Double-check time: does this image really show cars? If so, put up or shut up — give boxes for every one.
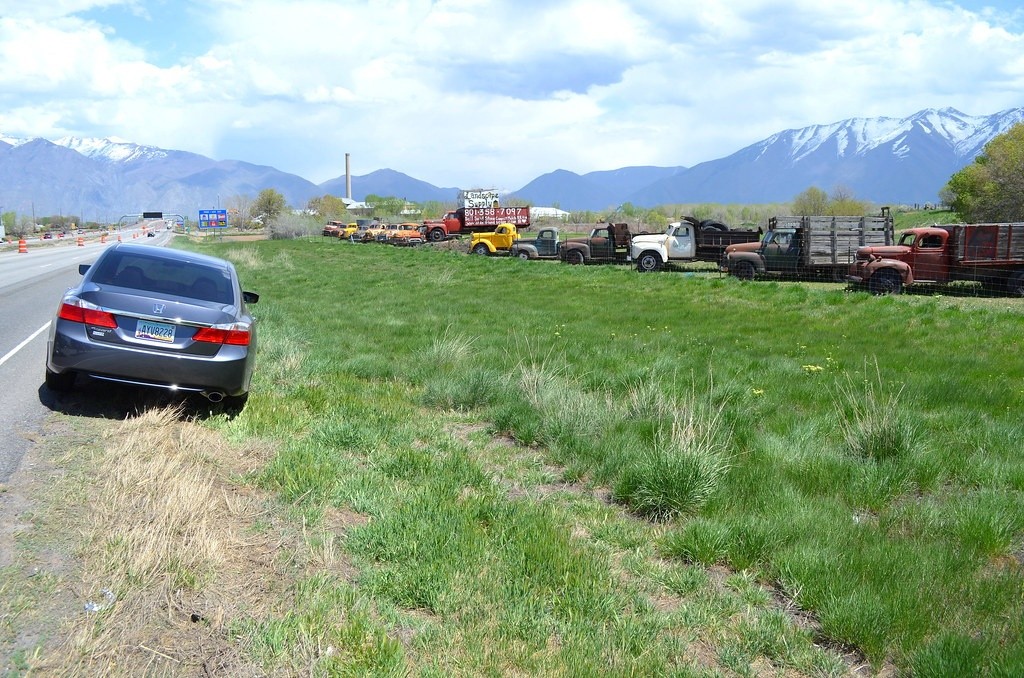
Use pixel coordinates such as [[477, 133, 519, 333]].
[[77, 230, 83, 234], [135, 221, 140, 224], [99, 226, 106, 231], [155, 229, 160, 232], [45, 241, 259, 416], [59, 232, 64, 237], [44, 233, 51, 239], [147, 232, 155, 237], [113, 226, 119, 230], [165, 219, 178, 229]]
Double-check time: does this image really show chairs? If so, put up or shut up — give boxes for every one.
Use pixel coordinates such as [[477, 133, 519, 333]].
[[114, 265, 146, 288], [187, 276, 218, 302]]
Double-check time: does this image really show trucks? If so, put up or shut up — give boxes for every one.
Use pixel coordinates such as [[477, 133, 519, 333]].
[[465, 223, 522, 257], [718, 207, 895, 283], [322, 221, 428, 246], [846, 223, 1024, 298], [508, 227, 563, 260], [626, 216, 764, 272], [423, 189, 531, 242], [558, 223, 666, 265]]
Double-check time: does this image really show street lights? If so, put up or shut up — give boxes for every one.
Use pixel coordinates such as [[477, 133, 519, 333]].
[[217, 192, 224, 242]]
